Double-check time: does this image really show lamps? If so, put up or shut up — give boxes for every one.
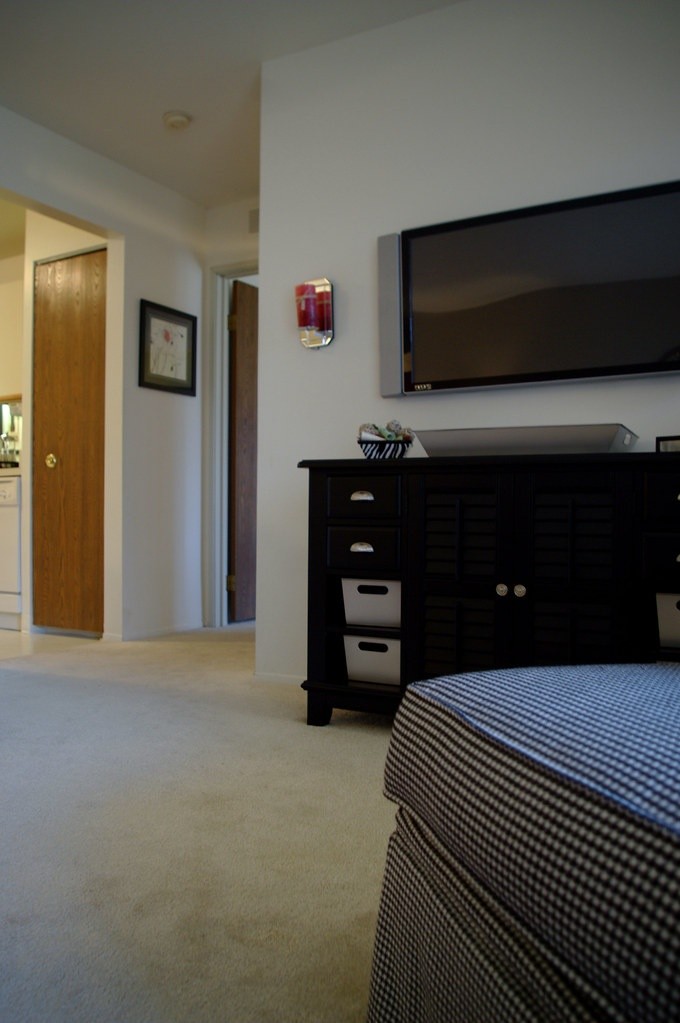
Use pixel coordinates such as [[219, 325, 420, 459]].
[[296, 277, 336, 350]]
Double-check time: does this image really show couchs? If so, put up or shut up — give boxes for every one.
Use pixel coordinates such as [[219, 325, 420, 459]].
[[362, 661, 680, 1023]]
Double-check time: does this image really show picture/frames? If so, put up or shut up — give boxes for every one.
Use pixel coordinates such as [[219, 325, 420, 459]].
[[138, 298, 198, 397]]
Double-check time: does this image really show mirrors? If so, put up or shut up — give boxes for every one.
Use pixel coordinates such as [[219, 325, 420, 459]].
[[0, 394, 22, 455]]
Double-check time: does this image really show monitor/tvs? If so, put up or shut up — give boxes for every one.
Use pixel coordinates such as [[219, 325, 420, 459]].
[[399, 179, 680, 396]]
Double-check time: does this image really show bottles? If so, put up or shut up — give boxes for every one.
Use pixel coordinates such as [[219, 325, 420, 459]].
[[0, 432, 19, 467]]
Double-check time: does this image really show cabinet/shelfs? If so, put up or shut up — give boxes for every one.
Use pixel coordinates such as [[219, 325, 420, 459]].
[[298, 453, 680, 727], [0, 476, 20, 631]]
